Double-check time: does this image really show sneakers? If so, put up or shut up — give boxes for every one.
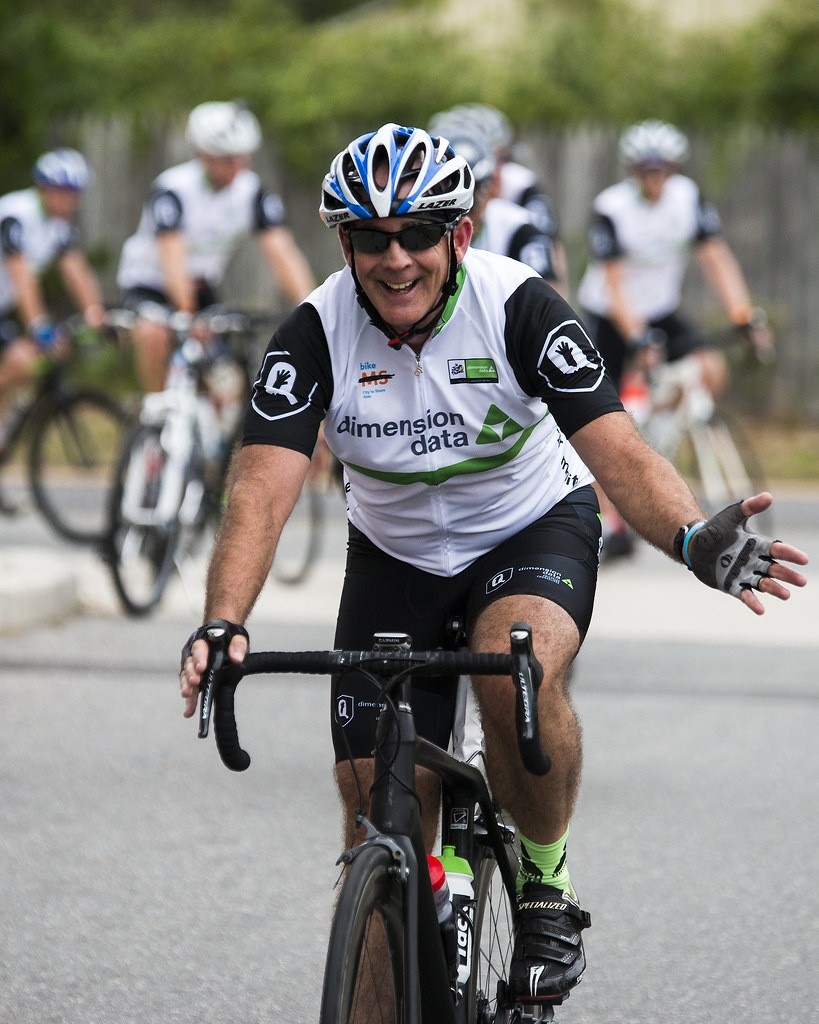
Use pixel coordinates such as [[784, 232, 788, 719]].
[[509, 880, 593, 1004]]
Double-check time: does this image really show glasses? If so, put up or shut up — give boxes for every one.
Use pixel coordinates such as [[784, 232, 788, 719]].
[[341, 213, 466, 256]]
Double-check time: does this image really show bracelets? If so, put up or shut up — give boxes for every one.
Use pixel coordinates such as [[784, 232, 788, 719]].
[[674, 518, 705, 567]]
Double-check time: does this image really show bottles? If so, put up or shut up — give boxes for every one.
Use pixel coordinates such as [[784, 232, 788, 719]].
[[422, 854, 462, 981], [432, 844, 474, 984]]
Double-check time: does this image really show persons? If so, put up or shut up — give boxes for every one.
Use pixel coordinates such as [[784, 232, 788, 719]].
[[0, 148, 103, 394], [578, 121, 767, 561], [115, 101, 318, 472], [429, 103, 570, 300], [178, 122, 808, 1024]]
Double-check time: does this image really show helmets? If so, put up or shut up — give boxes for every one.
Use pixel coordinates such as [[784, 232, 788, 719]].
[[318, 124, 474, 226], [187, 99, 262, 159], [424, 134, 498, 194], [31, 149, 91, 191], [617, 120, 691, 171], [453, 102, 517, 163]]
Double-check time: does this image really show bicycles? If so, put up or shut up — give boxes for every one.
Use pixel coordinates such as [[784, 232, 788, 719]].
[[104, 299, 284, 617], [196, 618, 569, 1023], [1, 333, 133, 546], [640, 320, 774, 542], [229, 314, 326, 585]]
